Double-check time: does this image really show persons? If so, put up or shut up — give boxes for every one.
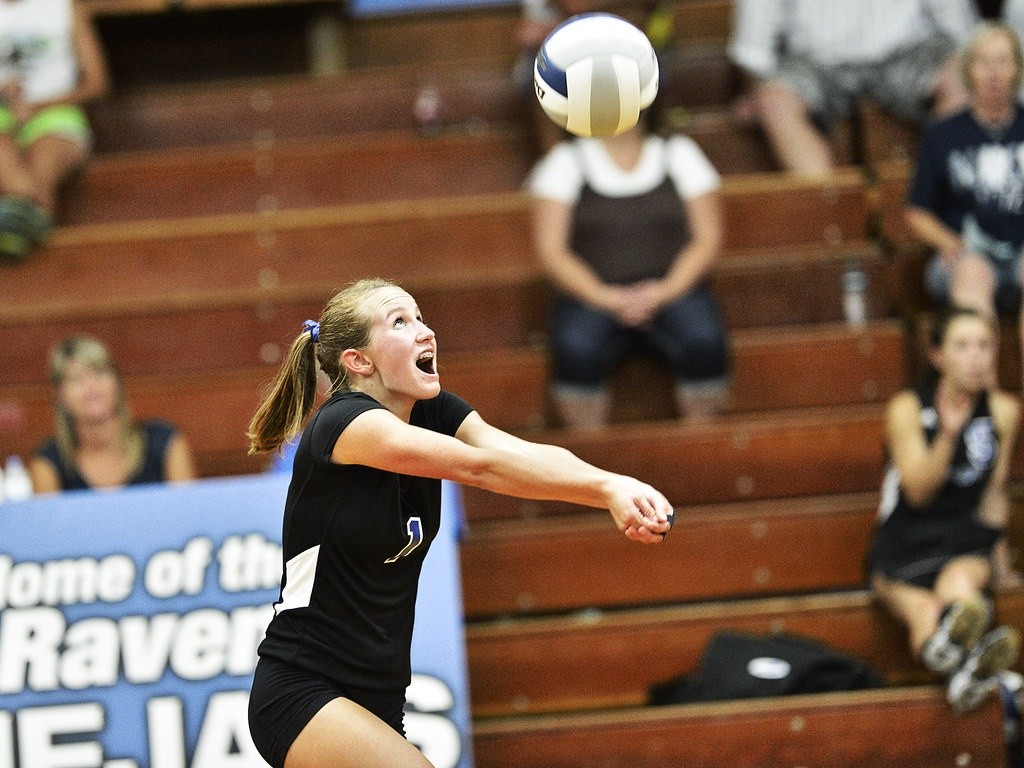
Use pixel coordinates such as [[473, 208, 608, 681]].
[[0, 0, 112, 264], [242, 272, 676, 768], [898, 22, 1024, 351], [24, 331, 200, 495], [861, 306, 1024, 715], [501, 0, 731, 432], [719, 0, 984, 173]]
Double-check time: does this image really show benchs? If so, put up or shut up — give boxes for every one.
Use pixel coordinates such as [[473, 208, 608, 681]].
[[0, 34, 1024, 768]]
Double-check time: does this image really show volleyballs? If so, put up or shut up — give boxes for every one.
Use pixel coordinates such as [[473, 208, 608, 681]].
[[531, 10, 660, 139]]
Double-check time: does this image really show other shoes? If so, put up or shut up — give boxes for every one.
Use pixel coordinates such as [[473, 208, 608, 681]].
[[947, 628, 1019, 713], [0, 196, 51, 258], [914, 596, 991, 675]]
